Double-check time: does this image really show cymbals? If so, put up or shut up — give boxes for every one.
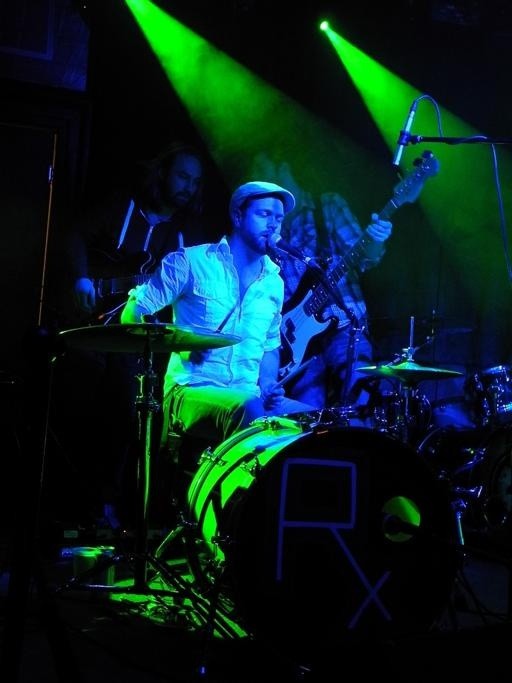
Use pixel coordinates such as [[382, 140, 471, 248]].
[[58, 323, 234, 353], [356, 362, 464, 381]]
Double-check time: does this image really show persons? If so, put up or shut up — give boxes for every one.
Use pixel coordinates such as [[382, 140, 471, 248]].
[[75, 148, 203, 312], [251, 147, 392, 427], [119, 179, 319, 444]]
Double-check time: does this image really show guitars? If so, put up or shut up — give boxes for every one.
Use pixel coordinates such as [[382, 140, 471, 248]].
[[29, 252, 152, 320], [280, 151, 440, 379]]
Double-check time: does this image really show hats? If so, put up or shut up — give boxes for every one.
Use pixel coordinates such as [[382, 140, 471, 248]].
[[229, 181, 296, 213]]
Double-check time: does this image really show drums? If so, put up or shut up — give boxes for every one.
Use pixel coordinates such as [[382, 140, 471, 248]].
[[375, 392, 479, 478], [187, 416, 419, 591], [464, 366, 511, 425]]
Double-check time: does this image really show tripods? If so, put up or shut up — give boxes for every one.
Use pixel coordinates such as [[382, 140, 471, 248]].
[[54, 348, 245, 638]]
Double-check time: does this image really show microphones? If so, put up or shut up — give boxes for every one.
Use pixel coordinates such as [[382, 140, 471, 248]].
[[393, 99, 419, 167], [382, 515, 432, 545], [267, 233, 321, 271]]
